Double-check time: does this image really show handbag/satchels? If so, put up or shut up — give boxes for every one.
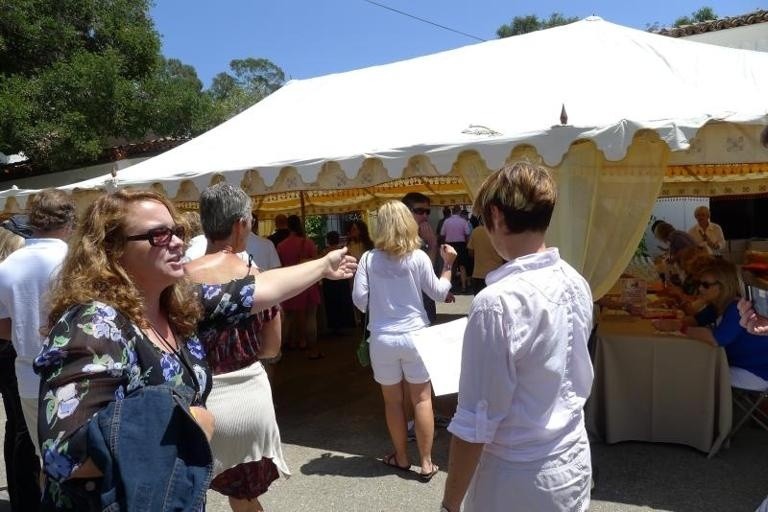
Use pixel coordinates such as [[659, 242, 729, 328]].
[[355, 338, 370, 366]]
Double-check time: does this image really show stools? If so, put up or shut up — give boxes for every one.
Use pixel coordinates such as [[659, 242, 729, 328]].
[[722, 365, 767, 445]]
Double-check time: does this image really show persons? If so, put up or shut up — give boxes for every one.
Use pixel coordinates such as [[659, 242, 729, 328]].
[[597, 205, 766, 402], [352, 194, 504, 480], [438, 159, 595, 512], [0, 182, 358, 511]]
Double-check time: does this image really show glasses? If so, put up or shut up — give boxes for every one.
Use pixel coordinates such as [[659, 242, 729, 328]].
[[696, 279, 717, 289], [117, 226, 186, 247], [412, 207, 431, 215]]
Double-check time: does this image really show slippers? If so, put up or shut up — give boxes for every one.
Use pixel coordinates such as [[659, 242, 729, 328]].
[[419, 465, 438, 480], [382, 455, 411, 469]]
